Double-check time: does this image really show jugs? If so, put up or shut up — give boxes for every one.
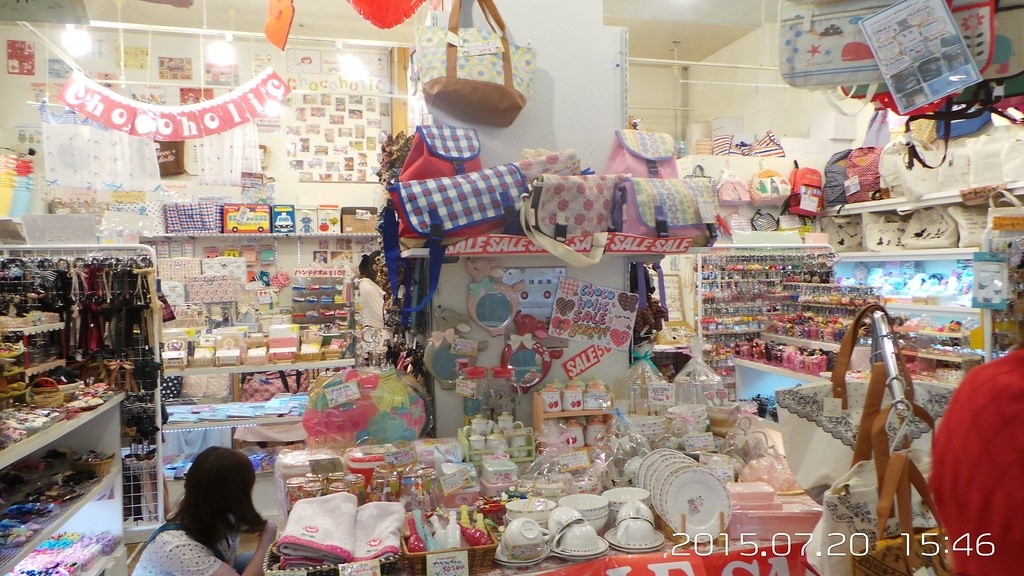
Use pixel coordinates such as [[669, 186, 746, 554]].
[[489, 366, 522, 414], [462, 366, 488, 415]]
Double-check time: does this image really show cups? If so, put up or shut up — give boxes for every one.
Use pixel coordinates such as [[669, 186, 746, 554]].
[[285, 465, 441, 511], [509, 435, 528, 458], [541, 375, 606, 411], [500, 500, 655, 559], [469, 435, 485, 462], [561, 417, 606, 447]]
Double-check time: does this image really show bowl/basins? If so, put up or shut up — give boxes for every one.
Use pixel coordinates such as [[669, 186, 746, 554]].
[[631, 403, 709, 446], [504, 487, 650, 530]]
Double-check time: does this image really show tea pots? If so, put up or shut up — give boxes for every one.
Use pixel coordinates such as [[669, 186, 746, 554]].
[[464, 414, 494, 439], [486, 429, 508, 457], [489, 411, 523, 434]]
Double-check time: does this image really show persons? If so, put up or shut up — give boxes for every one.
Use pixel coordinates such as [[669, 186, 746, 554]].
[[359, 251, 386, 326], [132, 447, 276, 576]]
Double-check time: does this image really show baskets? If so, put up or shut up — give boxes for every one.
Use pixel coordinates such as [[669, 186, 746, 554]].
[[29, 378, 63, 408], [400, 520, 498, 576], [70, 458, 112, 477], [262, 540, 402, 576]]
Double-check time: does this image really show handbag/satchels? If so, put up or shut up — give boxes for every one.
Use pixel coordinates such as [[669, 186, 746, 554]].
[[803, 362, 943, 576], [775, 303, 960, 506], [381, 0, 1024, 314], [239, 370, 308, 402], [850, 452, 954, 576]]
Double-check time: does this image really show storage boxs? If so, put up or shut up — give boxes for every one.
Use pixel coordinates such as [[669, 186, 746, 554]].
[[185, 275, 237, 304], [222, 203, 377, 232]]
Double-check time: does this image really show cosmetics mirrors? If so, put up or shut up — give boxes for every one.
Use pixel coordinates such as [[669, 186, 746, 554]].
[[423, 305, 488, 390], [501, 311, 562, 394], [465, 258, 525, 336]]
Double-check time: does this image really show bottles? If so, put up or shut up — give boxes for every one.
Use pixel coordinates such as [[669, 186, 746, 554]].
[[407, 505, 493, 552]]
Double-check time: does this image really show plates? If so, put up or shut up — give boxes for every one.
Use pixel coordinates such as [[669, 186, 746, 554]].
[[637, 449, 732, 542], [493, 526, 666, 567]]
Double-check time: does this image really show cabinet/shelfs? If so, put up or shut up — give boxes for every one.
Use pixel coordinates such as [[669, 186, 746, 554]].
[[733, 181, 1023, 419], [0, 388, 128, 576]]
